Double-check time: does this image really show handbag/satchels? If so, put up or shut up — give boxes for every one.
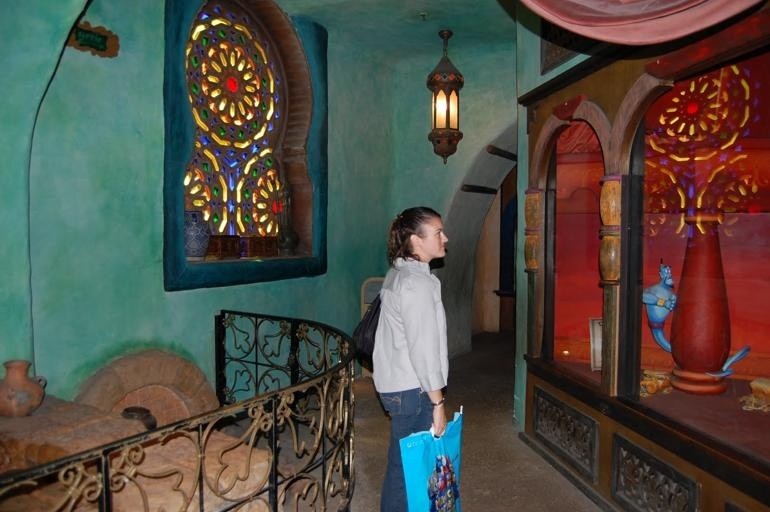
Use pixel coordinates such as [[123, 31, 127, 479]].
[[352, 292, 382, 373]]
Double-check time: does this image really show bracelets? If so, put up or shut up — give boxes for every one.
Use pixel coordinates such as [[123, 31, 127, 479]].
[[431, 397, 445, 406]]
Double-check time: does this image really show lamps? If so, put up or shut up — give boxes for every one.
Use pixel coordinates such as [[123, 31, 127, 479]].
[[427, 27, 464, 168]]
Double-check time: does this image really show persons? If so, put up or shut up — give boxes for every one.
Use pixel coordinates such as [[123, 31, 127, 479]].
[[373, 206, 449, 512]]
